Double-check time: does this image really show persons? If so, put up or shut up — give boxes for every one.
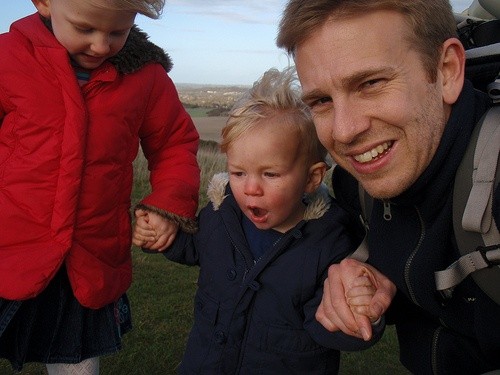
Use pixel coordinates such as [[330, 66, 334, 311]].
[[274, 0, 500, 375], [0, 0, 204, 375], [129, 62, 390, 374]]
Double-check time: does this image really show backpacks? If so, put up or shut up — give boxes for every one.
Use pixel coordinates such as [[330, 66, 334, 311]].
[[357, 2, 500, 309]]
